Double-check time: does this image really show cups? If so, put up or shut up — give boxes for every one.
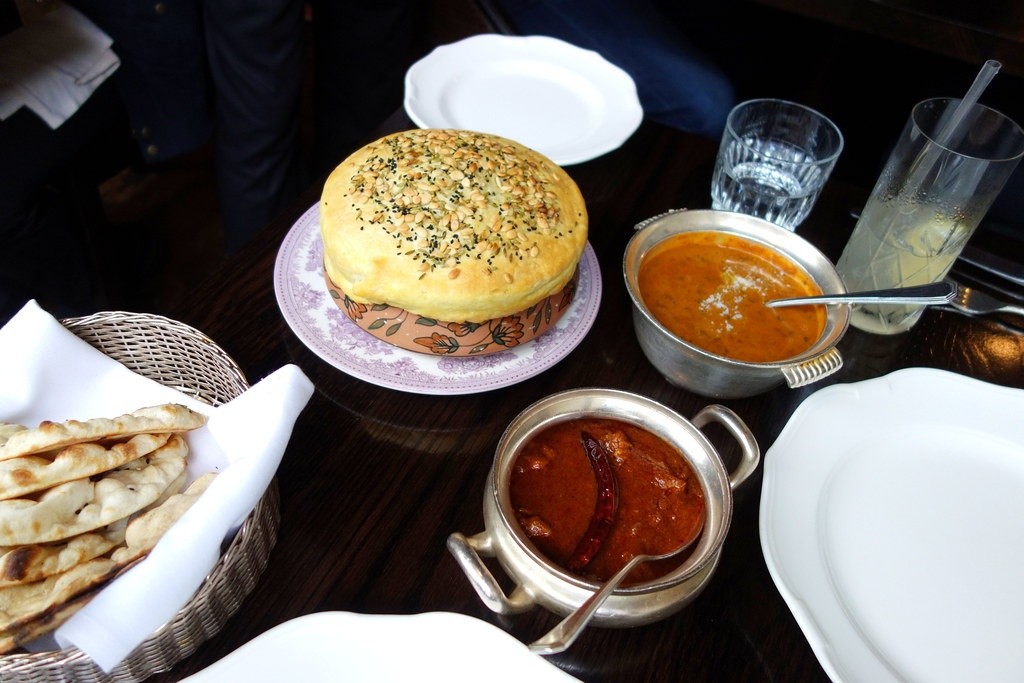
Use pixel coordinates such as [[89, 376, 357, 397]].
[[835, 96, 1024, 336], [709, 97, 845, 232]]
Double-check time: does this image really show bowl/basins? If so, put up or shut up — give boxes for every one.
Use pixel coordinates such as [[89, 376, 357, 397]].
[[621, 205, 853, 397], [445, 386, 763, 631]]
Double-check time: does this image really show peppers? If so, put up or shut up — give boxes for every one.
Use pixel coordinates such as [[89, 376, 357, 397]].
[[561, 431, 621, 575]]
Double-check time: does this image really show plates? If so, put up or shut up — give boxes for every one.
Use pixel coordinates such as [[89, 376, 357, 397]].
[[402, 32, 646, 166], [272, 199, 603, 396], [757, 366, 1024, 683], [173, 609, 583, 683]]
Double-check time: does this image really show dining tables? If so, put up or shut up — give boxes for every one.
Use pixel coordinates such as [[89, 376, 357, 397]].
[[140, 104, 1024, 683]]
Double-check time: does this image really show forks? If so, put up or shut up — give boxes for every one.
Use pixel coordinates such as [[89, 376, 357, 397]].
[[941, 276, 1024, 317]]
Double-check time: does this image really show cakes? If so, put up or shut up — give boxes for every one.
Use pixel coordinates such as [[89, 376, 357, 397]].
[[321, 130, 587, 358]]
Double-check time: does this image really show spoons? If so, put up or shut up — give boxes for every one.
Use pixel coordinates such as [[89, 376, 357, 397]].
[[528, 504, 708, 655]]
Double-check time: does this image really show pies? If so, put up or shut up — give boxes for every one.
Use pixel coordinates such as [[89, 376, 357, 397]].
[[0, 404, 215, 658]]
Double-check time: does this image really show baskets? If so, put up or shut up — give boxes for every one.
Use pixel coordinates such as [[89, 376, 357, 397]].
[[0, 311, 280, 683]]
